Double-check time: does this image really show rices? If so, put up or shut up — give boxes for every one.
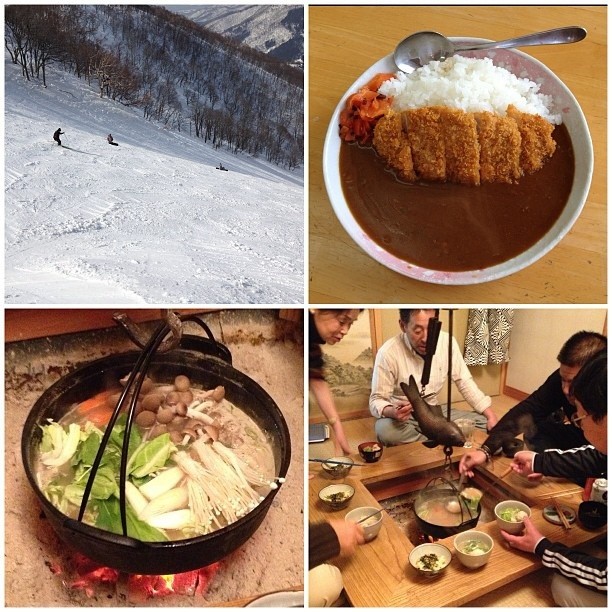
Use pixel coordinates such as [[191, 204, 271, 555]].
[[378, 34, 560, 124]]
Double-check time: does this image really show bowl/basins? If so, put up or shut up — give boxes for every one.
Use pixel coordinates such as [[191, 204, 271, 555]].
[[578, 501, 607, 530], [323, 36, 594, 287], [408, 543, 452, 579], [345, 506, 383, 541], [494, 500, 531, 533], [502, 438, 524, 458], [321, 456, 354, 479], [318, 483, 355, 511], [358, 442, 383, 463], [453, 531, 494, 570], [454, 419, 476, 434]]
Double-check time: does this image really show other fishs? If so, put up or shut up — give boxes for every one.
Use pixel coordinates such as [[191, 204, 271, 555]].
[[399, 374, 467, 449]]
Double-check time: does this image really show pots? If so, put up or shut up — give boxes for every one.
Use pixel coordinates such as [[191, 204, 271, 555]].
[[21, 311, 291, 575], [412, 476, 481, 539]]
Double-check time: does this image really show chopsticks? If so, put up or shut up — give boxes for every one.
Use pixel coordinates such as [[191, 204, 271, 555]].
[[308, 459, 367, 466], [357, 507, 387, 524], [551, 496, 572, 530], [395, 392, 436, 410]]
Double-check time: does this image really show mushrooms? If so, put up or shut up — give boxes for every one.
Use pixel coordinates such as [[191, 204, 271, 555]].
[[108, 373, 226, 447]]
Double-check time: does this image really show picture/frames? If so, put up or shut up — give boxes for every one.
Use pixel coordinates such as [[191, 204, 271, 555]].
[[309, 309, 377, 426]]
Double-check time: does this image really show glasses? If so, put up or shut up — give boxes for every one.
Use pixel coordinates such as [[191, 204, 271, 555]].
[[571, 412, 593, 428]]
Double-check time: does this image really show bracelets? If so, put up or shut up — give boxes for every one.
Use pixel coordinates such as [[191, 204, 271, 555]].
[[477, 448, 491, 463]]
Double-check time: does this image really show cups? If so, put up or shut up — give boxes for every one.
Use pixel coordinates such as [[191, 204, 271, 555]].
[[462, 419, 476, 448]]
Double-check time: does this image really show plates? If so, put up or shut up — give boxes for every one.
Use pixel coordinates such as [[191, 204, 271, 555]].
[[543, 504, 577, 525]]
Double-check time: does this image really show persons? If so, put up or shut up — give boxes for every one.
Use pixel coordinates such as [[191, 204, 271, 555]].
[[500, 348, 607, 606], [309, 309, 367, 457], [107, 133, 113, 143], [369, 309, 500, 447], [307, 519, 367, 607], [458, 330, 607, 483], [53, 128, 64, 146]]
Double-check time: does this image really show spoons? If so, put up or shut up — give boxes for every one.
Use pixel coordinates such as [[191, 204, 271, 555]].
[[444, 455, 471, 514], [461, 466, 513, 503], [393, 26, 587, 74]]
